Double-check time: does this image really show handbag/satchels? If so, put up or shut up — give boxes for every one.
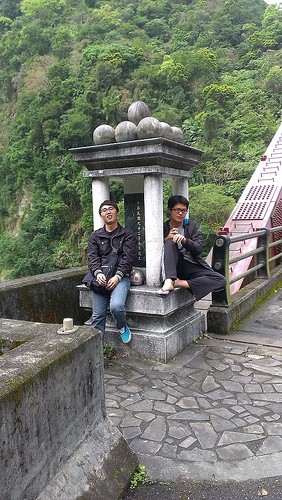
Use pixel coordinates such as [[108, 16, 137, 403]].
[[82, 253, 117, 295]]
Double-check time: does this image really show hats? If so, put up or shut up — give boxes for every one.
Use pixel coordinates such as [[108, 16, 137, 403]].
[[98, 200, 119, 215]]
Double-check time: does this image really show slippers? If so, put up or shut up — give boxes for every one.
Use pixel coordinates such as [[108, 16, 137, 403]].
[[155, 285, 175, 298]]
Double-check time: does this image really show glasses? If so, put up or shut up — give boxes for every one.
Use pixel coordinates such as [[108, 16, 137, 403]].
[[173, 208, 190, 213]]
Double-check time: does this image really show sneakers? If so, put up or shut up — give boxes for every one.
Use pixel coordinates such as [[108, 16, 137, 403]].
[[118, 325, 132, 343]]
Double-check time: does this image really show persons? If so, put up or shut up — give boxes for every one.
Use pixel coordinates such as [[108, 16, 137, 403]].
[[157, 195, 227, 301], [86, 200, 136, 344]]
